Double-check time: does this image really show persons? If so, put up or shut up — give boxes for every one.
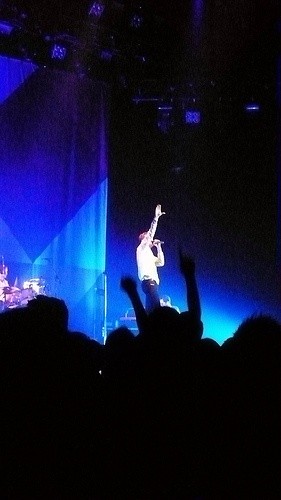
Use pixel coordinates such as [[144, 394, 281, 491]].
[[0, 264, 10, 302], [119, 245, 281, 500], [0, 295, 136, 500], [136, 204, 166, 313]]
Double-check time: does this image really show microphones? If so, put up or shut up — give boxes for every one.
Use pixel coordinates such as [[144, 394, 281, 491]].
[[151, 240, 165, 244]]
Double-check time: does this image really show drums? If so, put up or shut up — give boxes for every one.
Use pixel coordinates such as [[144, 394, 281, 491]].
[[21, 288, 36, 305], [4, 286, 20, 308]]
